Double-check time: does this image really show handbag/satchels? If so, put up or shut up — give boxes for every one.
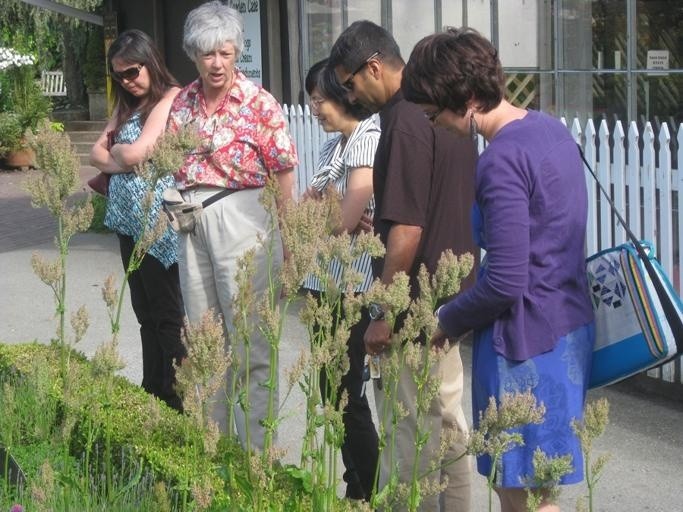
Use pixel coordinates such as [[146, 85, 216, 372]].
[[586, 234, 682, 391], [161, 187, 202, 233], [87, 131, 113, 196]]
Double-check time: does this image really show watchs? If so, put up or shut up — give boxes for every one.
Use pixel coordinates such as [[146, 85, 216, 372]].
[[368, 302, 385, 321]]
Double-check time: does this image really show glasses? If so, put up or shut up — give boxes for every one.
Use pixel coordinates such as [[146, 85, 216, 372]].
[[425, 107, 446, 126], [340, 51, 379, 91], [110, 63, 143, 82]]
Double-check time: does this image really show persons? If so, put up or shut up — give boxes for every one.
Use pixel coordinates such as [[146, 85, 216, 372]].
[[300, 59, 381, 499], [329, 19, 480, 512], [401, 26, 596, 512], [165, 0, 296, 467], [87, 27, 187, 416]]
